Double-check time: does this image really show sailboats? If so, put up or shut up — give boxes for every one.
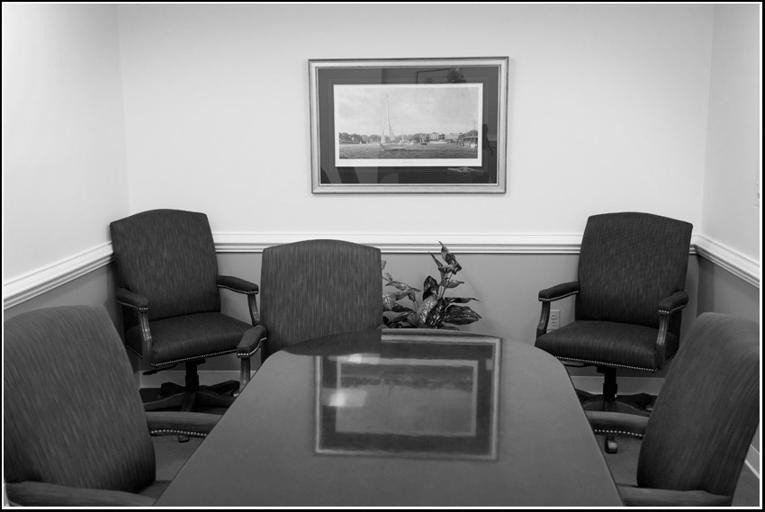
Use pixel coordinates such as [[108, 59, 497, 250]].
[[379, 92, 433, 151]]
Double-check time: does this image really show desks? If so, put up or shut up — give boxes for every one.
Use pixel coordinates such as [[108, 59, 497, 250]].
[[151, 329, 625, 506]]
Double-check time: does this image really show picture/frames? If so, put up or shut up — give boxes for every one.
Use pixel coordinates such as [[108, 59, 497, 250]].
[[308, 56, 508, 197], [312, 332, 503, 461]]
